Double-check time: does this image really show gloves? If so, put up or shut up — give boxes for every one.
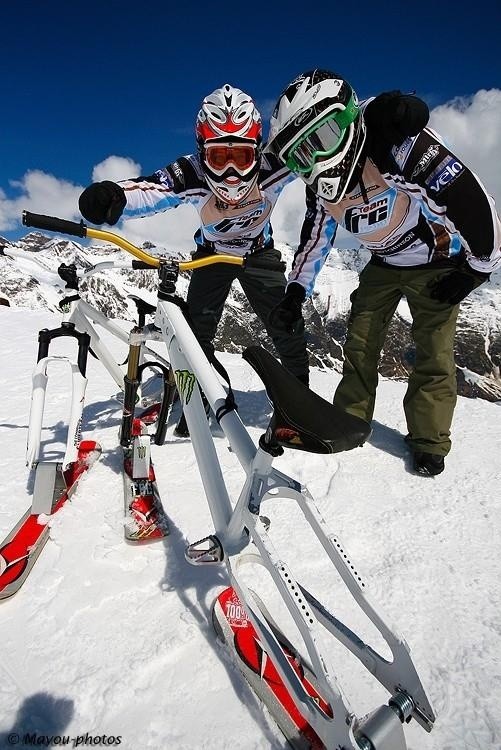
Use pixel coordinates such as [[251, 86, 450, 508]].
[[266, 281, 305, 338], [78, 181, 125, 226], [362, 88, 428, 147], [426, 260, 490, 305]]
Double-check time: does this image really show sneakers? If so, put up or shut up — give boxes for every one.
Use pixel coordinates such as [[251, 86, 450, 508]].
[[409, 445, 445, 476], [174, 390, 211, 437]]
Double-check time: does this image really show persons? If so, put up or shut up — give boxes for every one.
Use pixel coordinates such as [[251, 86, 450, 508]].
[[77, 85, 430, 437], [264, 67, 501, 478]]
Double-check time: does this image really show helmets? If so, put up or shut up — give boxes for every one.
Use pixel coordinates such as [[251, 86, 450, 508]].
[[259, 68, 367, 205], [194, 83, 263, 207]]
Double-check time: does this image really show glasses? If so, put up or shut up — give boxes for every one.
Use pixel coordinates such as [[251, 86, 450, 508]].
[[284, 91, 359, 174], [199, 141, 261, 182]]
